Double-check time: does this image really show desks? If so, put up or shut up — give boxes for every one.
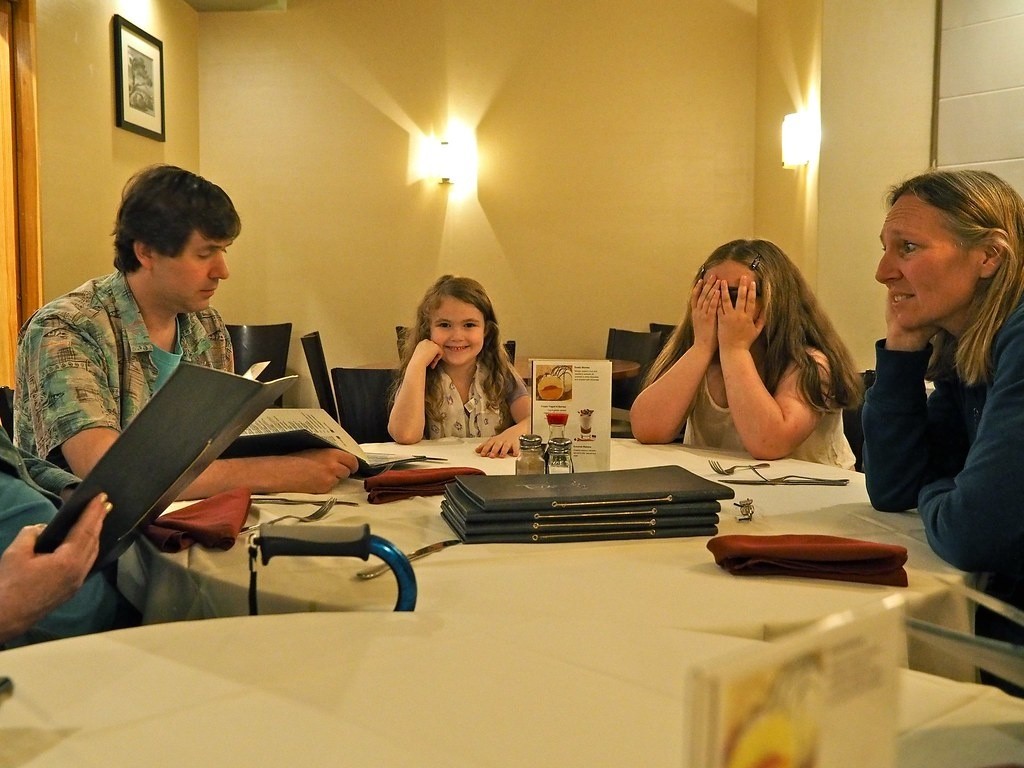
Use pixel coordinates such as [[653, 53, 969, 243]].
[[0, 607, 1024, 768], [514, 354, 645, 407]]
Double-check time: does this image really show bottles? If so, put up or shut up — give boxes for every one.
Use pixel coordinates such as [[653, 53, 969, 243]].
[[515, 434, 545, 475], [544, 423, 575, 474]]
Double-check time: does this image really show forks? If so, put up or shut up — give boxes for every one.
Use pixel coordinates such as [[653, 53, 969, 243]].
[[749, 465, 850, 483], [238, 498, 337, 536], [708, 460, 771, 475]]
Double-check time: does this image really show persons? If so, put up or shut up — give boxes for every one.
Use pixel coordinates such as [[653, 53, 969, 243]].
[[0, 424, 121, 654], [860, 170, 1023, 701], [12, 165, 359, 501], [629, 240, 864, 472], [388, 276, 532, 458]]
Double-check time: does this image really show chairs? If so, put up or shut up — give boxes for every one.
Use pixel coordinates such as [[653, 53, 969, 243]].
[[648, 322, 677, 353], [606, 326, 664, 412], [223, 321, 294, 409], [299, 331, 339, 427], [328, 366, 404, 444], [394, 327, 519, 368]]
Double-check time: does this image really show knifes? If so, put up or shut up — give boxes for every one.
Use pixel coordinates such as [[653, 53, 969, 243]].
[[356, 539, 463, 580], [718, 480, 847, 486], [248, 497, 359, 507]]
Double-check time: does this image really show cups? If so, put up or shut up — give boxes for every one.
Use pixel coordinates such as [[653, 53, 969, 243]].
[[579, 414, 592, 437]]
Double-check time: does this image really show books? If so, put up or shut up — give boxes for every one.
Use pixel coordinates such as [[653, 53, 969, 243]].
[[440, 465, 734, 544], [34, 361, 426, 583]]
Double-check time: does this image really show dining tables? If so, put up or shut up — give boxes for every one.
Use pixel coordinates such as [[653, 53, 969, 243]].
[[129, 431, 979, 684]]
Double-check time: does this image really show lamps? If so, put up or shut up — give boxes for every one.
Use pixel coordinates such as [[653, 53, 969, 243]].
[[428, 139, 462, 186], [781, 112, 809, 170]]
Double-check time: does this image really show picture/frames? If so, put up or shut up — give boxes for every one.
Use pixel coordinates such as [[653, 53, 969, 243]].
[[113, 13, 167, 145]]
